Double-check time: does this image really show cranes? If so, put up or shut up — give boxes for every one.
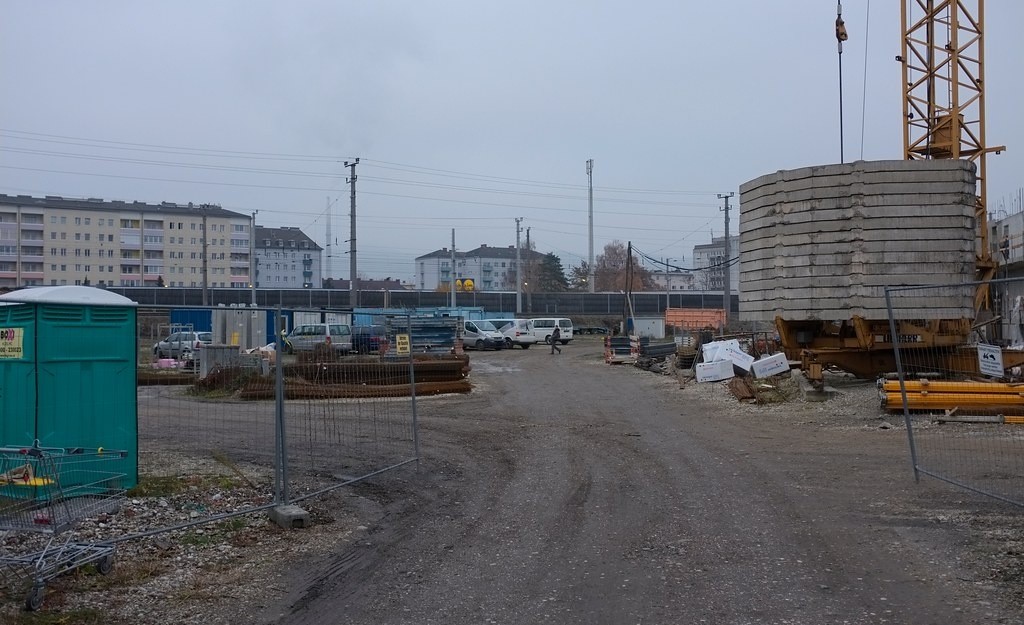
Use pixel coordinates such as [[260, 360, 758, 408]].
[[769, 0, 1024, 386]]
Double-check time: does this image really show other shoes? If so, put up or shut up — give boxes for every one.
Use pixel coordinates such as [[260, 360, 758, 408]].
[[559, 349, 561, 354], [550, 352, 554, 354]]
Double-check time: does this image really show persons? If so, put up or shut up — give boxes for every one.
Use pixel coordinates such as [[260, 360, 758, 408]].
[[548, 324, 562, 354]]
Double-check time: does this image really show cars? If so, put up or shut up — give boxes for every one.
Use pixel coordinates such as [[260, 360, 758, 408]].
[[153, 330, 213, 360]]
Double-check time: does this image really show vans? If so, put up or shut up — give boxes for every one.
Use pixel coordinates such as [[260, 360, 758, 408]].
[[485, 319, 536, 350], [284, 323, 353, 357], [462, 320, 506, 352], [350, 324, 386, 354], [531, 318, 574, 345]]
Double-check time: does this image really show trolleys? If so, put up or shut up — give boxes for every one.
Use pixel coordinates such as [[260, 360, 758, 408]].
[[1, 437, 128, 611]]
